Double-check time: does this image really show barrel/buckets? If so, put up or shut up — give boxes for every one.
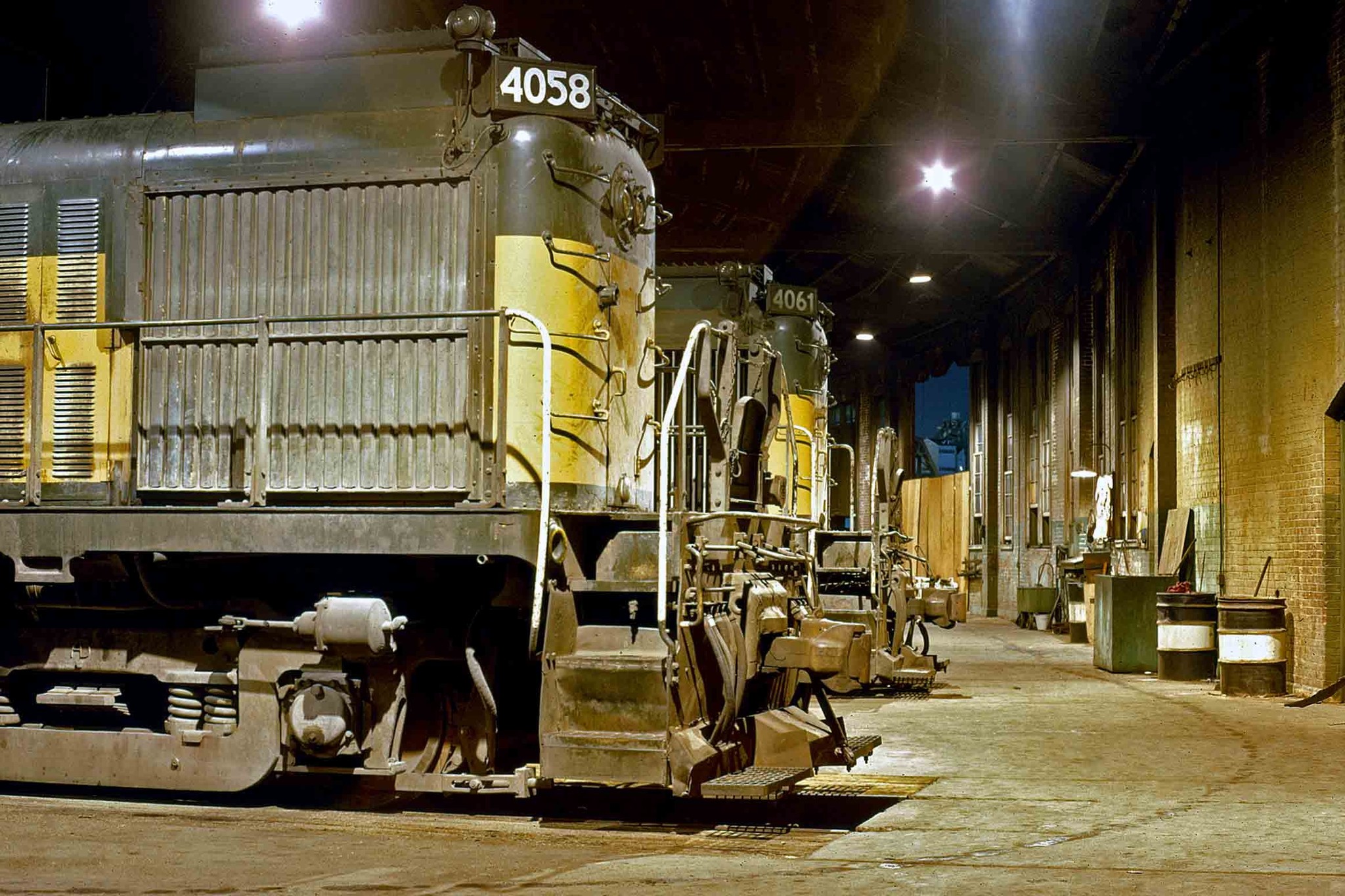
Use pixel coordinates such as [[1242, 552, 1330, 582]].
[[1155, 592, 1219, 681], [1216, 595, 1289, 697], [1035, 614, 1050, 631]]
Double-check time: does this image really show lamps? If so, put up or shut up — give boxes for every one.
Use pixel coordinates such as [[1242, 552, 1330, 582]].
[[856, 311, 874, 341], [909, 251, 931, 283]]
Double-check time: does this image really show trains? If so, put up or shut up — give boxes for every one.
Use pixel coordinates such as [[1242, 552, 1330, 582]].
[[0, 2, 969, 802]]
